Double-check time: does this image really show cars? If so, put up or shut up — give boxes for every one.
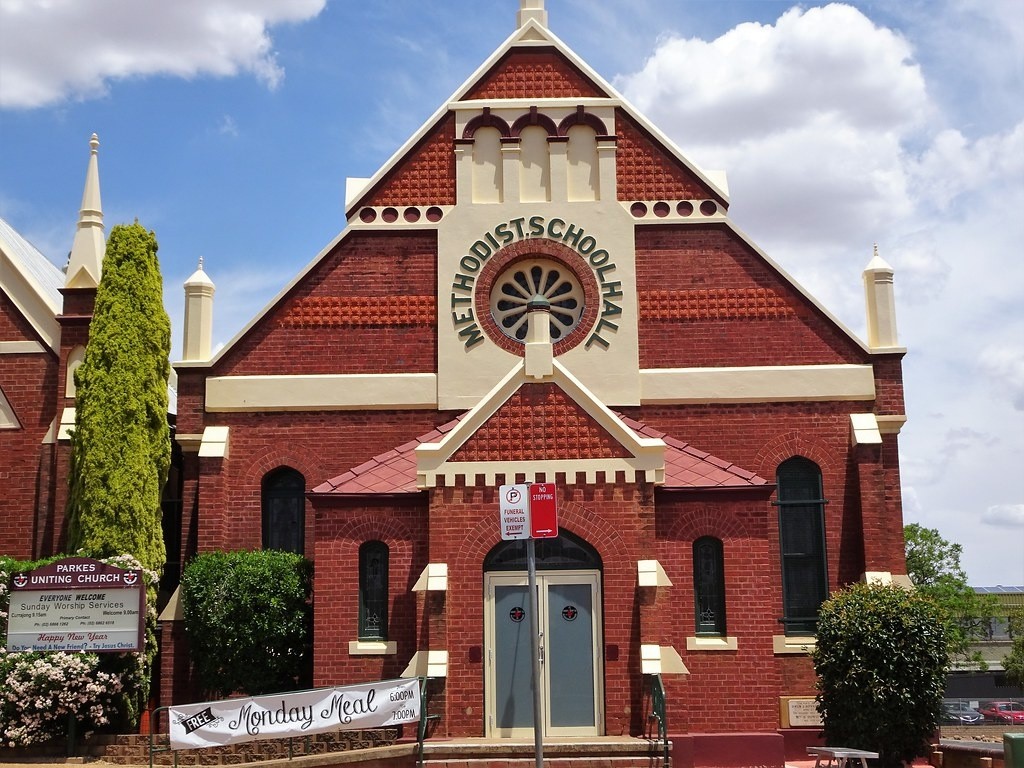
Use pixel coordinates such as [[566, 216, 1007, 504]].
[[976, 700, 1024, 725], [939, 701, 985, 726]]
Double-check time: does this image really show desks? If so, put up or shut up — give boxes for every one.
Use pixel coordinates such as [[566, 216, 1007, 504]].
[[806, 747, 879, 768]]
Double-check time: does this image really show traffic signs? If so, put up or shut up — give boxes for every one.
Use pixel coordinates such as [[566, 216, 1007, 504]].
[[499, 484, 531, 541], [529, 482, 558, 538]]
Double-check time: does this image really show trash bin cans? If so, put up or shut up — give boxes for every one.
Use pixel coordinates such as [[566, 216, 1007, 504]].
[[1002, 732, 1024, 768]]
